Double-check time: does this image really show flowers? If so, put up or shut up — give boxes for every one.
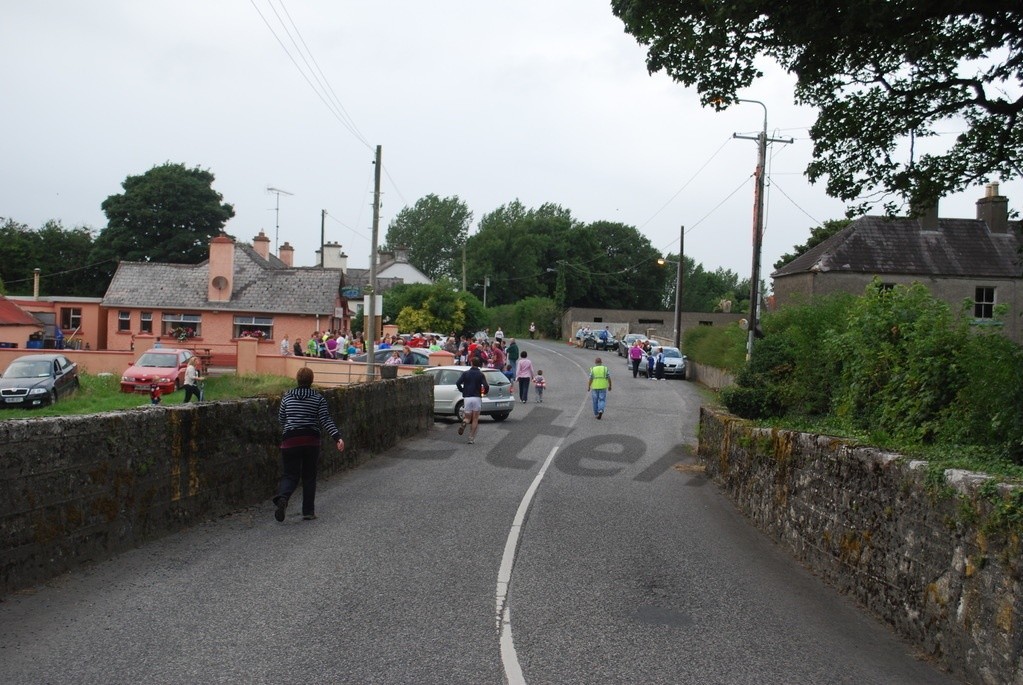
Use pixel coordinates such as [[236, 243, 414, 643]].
[[240, 330, 268, 337]]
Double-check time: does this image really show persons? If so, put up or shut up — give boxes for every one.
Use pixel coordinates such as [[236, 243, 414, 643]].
[[576, 325, 665, 381], [280, 322, 546, 404], [130, 335, 135, 351], [184, 357, 205, 403], [456, 357, 490, 444], [272, 367, 344, 522], [154, 337, 163, 348], [149, 376, 162, 405], [587, 358, 612, 419]]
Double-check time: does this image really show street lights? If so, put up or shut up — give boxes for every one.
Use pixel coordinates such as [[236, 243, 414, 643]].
[[657, 258, 682, 346]]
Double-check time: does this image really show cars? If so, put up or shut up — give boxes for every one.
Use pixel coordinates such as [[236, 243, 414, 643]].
[[118, 348, 201, 395], [0, 354, 80, 408], [584, 330, 621, 350], [350, 348, 458, 367], [619, 334, 689, 379], [419, 367, 518, 422]]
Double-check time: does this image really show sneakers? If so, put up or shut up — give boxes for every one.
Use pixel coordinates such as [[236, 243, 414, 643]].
[[467, 437, 477, 444], [597, 410, 603, 419], [458, 422, 466, 435]]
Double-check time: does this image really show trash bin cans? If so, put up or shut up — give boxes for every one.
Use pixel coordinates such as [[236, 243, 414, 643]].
[[26, 335, 58, 349]]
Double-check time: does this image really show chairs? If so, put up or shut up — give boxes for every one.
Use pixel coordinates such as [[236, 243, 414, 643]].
[[446, 372, 458, 385]]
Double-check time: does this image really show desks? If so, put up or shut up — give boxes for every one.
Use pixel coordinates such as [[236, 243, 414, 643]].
[[185, 348, 212, 366]]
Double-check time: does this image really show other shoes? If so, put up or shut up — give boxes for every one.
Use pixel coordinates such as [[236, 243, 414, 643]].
[[274, 498, 288, 522], [303, 515, 317, 520]]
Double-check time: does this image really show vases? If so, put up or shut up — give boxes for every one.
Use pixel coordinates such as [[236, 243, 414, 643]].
[[241, 336, 267, 340]]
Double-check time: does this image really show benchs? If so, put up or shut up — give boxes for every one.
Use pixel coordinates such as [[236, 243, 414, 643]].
[[195, 355, 214, 375]]
